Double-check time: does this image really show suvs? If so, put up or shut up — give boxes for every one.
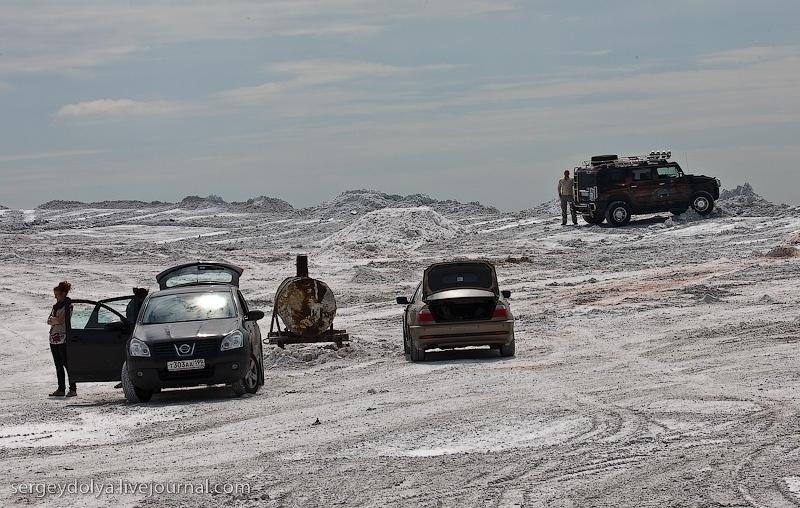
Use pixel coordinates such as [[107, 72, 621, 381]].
[[569, 150, 721, 227], [64, 259, 264, 402]]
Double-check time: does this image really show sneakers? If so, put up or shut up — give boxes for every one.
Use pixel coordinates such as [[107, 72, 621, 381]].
[[48, 388, 66, 396], [65, 389, 78, 397]]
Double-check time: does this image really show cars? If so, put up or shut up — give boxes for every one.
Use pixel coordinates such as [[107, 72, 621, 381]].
[[395, 260, 516, 361]]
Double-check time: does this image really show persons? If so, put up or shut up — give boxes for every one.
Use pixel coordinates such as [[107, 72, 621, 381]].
[[47, 280, 77, 397], [124, 287, 148, 325], [557, 170, 578, 226]]
